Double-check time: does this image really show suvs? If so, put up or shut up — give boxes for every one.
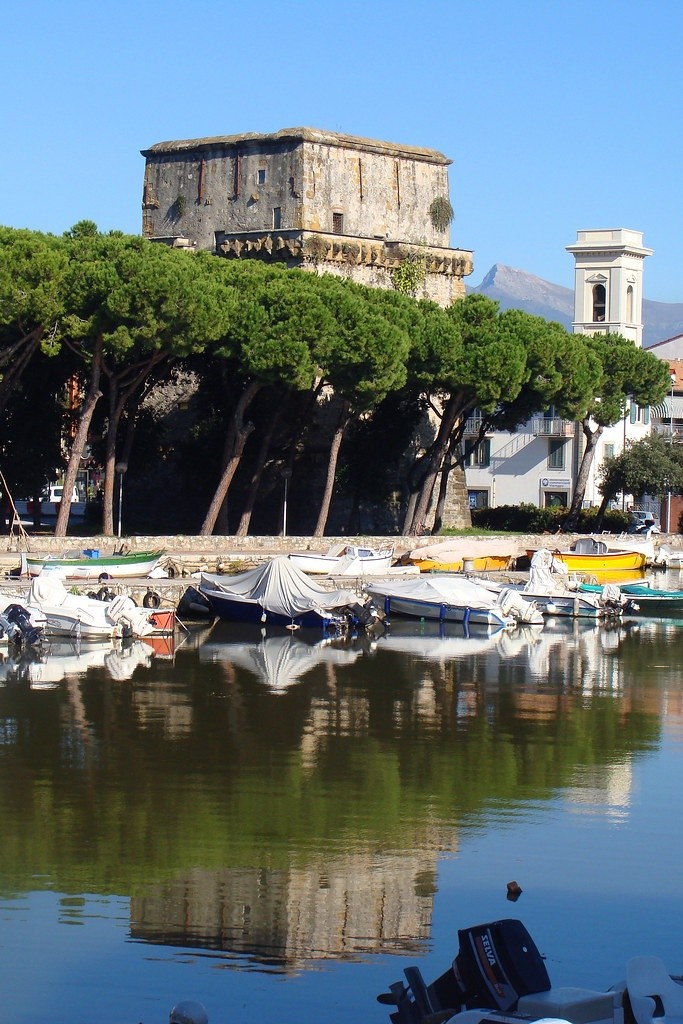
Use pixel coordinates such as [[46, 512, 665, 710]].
[[631, 511, 661, 534]]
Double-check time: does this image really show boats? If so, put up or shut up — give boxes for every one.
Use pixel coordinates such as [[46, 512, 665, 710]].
[[24, 634, 150, 688], [27, 580, 175, 640], [362, 578, 543, 626], [578, 581, 683, 608], [142, 635, 188, 660], [525, 538, 646, 572], [402, 539, 516, 573], [20, 546, 166, 579], [286, 540, 394, 574], [197, 574, 377, 631], [0, 595, 48, 647], [202, 618, 360, 691], [667, 552, 683, 569], [469, 577, 626, 619], [377, 616, 501, 662]]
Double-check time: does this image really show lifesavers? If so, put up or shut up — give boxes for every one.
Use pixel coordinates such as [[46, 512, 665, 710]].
[[163, 565, 178, 579], [87, 586, 117, 602], [143, 592, 160, 609]]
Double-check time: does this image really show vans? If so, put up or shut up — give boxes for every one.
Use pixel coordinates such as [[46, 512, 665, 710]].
[[31, 486, 79, 502]]
